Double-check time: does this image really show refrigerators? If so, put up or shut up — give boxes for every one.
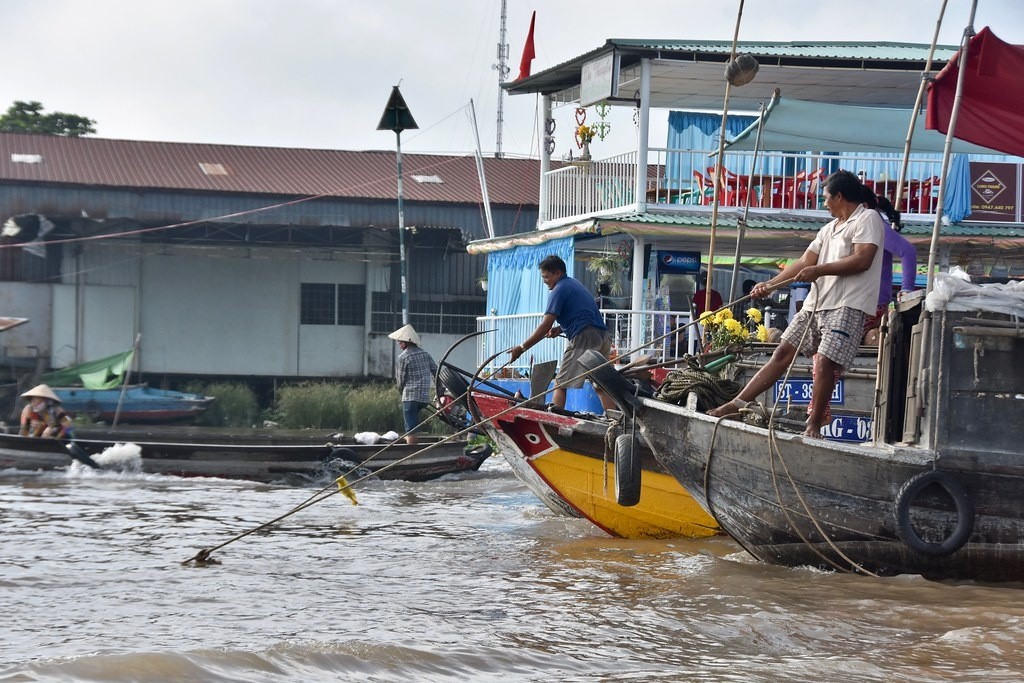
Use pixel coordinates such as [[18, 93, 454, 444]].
[[643, 252, 702, 357]]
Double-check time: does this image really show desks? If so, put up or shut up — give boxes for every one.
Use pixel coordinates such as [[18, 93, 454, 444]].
[[740, 174, 786, 208], [876, 179, 926, 212]]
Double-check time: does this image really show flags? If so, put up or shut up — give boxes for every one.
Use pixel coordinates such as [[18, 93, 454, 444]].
[[515, 10, 536, 83]]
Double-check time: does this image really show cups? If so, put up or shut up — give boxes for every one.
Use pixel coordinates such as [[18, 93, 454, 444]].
[[880, 173, 885, 181], [503, 368, 507, 377]]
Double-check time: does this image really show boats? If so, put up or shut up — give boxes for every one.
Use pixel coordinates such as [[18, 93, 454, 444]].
[[0, 421, 493, 480], [12, 382, 218, 423], [434, 297, 1023, 581]]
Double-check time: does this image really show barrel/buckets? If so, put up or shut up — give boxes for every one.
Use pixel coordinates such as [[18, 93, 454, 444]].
[[0, 373, 17, 422], [435, 395, 461, 419]]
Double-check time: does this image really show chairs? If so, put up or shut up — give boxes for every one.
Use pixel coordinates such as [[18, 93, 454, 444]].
[[692, 163, 940, 214]]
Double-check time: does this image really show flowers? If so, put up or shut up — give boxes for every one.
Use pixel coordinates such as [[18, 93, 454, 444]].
[[700, 307, 771, 350]]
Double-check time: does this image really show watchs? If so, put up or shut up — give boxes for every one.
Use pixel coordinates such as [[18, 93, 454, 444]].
[[520, 344, 526, 352]]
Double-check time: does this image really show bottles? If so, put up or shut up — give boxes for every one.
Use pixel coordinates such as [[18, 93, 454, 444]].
[[509, 369, 519, 378], [646, 279, 652, 347], [486, 370, 490, 378], [664, 176, 668, 189], [524, 371, 528, 378], [514, 390, 520, 399], [568, 149, 591, 161], [858, 169, 867, 180]]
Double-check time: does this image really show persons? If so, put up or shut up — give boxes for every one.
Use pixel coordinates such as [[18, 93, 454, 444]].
[[18, 384, 76, 439], [706, 170, 885, 441], [506, 254, 618, 412], [388, 324, 438, 444], [669, 186, 918, 356]]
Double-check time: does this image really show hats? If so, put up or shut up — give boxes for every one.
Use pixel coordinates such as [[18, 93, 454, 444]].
[[20, 383, 63, 403], [388, 323, 423, 347]]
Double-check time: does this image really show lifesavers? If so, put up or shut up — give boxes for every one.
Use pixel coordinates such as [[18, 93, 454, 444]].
[[893, 469, 976, 554], [438, 366, 478, 416], [614, 434, 642, 507], [466, 444, 492, 458], [576, 349, 636, 419]]
